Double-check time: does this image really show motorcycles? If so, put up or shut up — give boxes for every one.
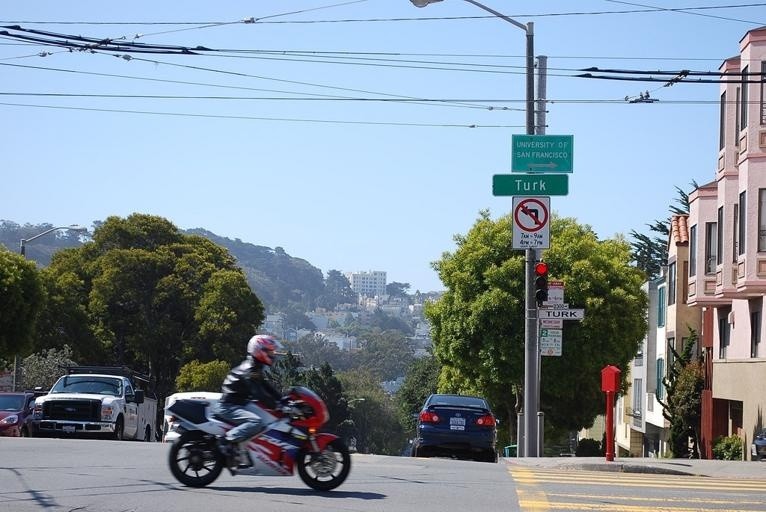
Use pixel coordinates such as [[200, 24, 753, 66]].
[[164, 385, 351, 491]]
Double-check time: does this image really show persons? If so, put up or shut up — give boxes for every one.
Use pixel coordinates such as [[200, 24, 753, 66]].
[[210, 333, 300, 455]]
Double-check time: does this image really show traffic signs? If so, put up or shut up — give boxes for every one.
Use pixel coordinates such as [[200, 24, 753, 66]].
[[511, 134, 574, 173]]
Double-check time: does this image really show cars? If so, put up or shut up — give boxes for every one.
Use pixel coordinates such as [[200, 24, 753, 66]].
[[416, 394, 496, 461], [0, 392, 34, 437]]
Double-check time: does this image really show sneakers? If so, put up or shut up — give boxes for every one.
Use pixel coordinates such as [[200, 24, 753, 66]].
[[215, 435, 235, 457]]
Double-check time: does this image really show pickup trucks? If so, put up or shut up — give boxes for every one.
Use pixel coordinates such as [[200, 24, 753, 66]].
[[32, 366, 158, 442]]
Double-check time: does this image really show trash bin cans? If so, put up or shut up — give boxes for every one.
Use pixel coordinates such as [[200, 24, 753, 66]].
[[504, 445, 517, 457]]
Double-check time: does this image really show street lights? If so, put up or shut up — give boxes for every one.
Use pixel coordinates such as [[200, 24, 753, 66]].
[[20, 224, 87, 256], [409, 0, 539, 457]]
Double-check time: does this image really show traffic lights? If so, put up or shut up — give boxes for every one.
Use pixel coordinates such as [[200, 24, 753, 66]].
[[533, 261, 548, 307]]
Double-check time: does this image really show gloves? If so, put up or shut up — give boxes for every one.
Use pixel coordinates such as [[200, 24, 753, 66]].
[[278, 404, 302, 417]]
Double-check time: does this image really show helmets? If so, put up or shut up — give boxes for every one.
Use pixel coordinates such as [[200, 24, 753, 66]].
[[245, 334, 285, 366]]
